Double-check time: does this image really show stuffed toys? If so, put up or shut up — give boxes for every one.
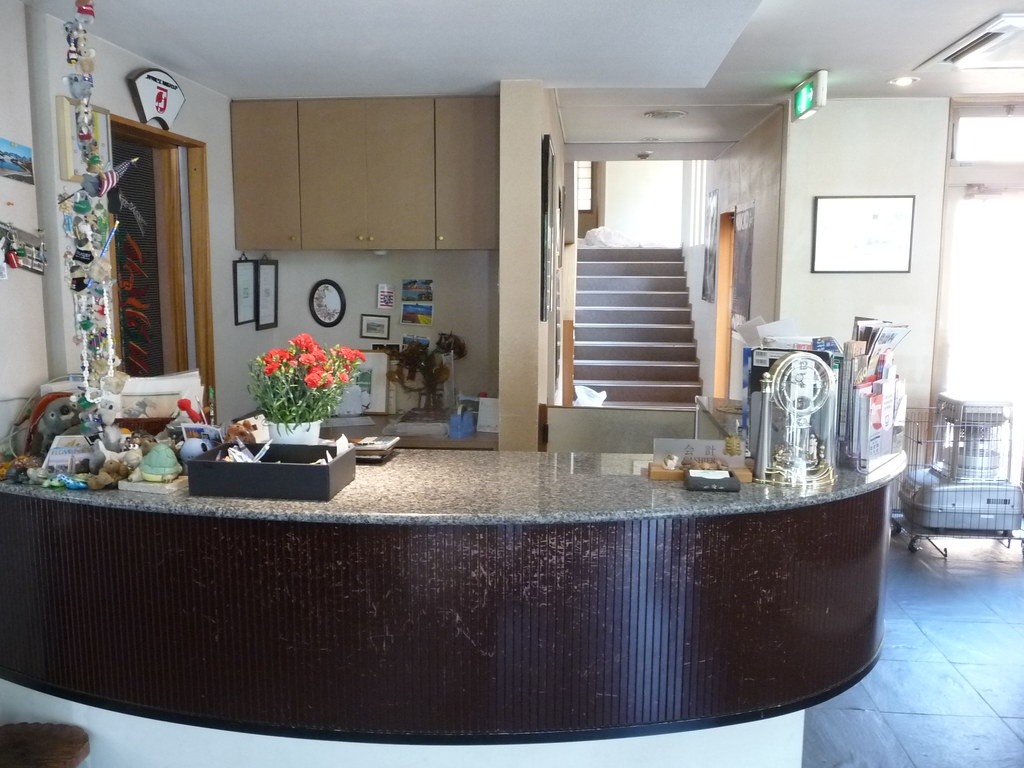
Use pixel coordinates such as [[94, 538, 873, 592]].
[[62, 0, 101, 297], [26, 305, 256, 494]]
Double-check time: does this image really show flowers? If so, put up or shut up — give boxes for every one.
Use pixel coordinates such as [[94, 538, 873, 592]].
[[247, 333, 366, 437]]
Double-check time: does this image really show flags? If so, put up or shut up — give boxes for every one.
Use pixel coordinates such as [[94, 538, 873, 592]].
[[99, 160, 133, 194]]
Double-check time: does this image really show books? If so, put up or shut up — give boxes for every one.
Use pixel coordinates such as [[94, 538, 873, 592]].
[[348, 433, 403, 468], [740, 316, 913, 469]]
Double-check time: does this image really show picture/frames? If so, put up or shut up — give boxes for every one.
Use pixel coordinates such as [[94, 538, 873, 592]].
[[400, 303, 434, 326], [256, 260, 278, 331], [56, 96, 114, 184], [811, 195, 915, 273], [309, 279, 346, 327], [371, 343, 401, 363], [360, 314, 390, 340], [541, 134, 567, 398], [232, 259, 257, 326]]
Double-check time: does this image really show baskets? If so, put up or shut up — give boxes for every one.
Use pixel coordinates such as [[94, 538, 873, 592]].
[[114, 417, 176, 437]]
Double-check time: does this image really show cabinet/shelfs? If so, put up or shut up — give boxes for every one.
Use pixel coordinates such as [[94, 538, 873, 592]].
[[435, 98, 499, 250], [230, 100, 301, 251], [297, 97, 435, 250]]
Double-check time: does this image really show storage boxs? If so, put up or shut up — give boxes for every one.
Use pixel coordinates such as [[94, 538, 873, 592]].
[[187, 442, 356, 501]]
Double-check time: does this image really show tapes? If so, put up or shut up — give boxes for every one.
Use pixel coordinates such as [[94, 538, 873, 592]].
[[452, 403, 473, 437]]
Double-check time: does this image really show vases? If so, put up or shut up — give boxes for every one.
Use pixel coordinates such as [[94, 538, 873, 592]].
[[267, 419, 324, 446]]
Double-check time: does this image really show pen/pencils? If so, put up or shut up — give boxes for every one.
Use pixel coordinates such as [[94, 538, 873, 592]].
[[192, 384, 220, 424]]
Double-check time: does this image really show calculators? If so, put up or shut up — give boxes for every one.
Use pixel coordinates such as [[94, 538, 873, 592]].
[[351, 433, 401, 451]]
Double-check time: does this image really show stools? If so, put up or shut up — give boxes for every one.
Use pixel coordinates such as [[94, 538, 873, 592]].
[[0, 722, 90, 768]]
